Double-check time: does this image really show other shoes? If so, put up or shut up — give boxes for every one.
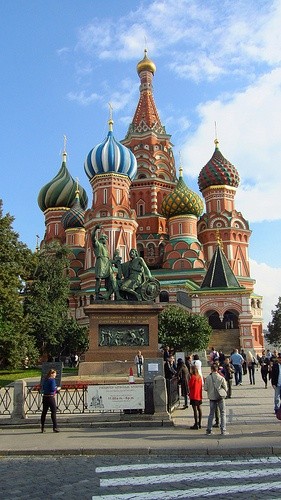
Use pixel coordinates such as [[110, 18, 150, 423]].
[[52, 428, 60, 432], [220, 430, 228, 435], [212, 424, 218, 428], [205, 431, 213, 435], [198, 424, 201, 429], [40, 428, 47, 433], [189, 425, 198, 430]]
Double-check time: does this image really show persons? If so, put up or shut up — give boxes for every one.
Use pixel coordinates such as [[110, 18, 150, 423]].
[[90, 224, 155, 302], [40, 368, 61, 433], [48, 345, 281, 436]]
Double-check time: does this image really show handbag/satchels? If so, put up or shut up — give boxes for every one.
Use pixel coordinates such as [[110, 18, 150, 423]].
[[214, 387, 227, 399]]
